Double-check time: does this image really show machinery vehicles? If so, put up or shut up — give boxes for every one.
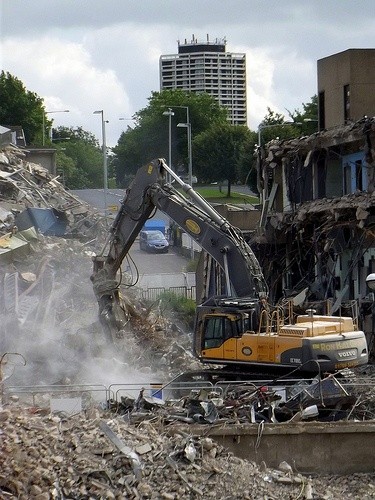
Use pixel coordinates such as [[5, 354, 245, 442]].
[[87, 156, 369, 421]]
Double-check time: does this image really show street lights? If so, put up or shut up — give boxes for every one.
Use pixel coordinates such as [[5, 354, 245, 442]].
[[163, 109, 173, 184], [160, 105, 191, 185], [176, 122, 192, 187], [258, 122, 304, 150], [92, 110, 108, 206], [41, 110, 70, 145]]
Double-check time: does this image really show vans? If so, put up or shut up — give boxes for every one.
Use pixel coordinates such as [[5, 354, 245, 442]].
[[139, 230, 170, 253]]
